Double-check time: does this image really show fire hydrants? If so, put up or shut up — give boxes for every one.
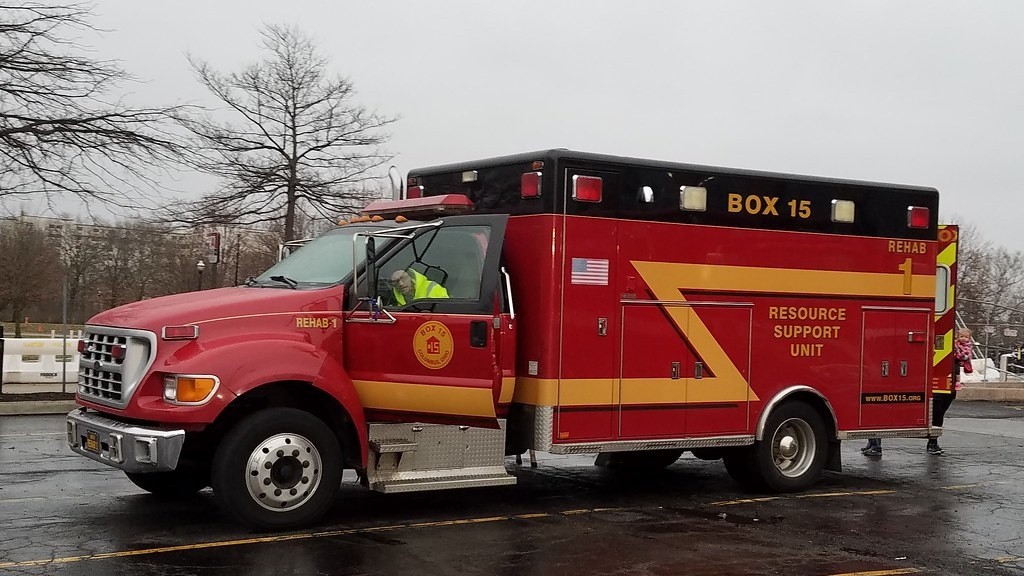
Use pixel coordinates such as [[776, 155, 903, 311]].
[[38, 323, 42, 332]]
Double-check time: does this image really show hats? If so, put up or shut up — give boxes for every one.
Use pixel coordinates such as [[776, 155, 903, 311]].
[[386, 264, 414, 290]]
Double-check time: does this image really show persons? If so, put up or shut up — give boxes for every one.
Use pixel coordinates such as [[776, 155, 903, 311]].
[[953, 329, 973, 391], [927, 390, 956, 454], [385, 266, 450, 308], [861, 437, 883, 456]]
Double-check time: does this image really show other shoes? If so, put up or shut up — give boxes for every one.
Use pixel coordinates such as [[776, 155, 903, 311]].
[[861, 443, 871, 451], [927, 444, 945, 454], [955, 383, 961, 390], [863, 445, 882, 456]]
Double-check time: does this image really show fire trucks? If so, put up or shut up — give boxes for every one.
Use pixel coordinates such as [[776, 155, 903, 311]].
[[66, 148, 959, 526]]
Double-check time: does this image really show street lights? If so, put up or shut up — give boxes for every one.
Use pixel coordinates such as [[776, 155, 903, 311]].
[[978, 317, 1003, 382], [195, 260, 207, 291]]
